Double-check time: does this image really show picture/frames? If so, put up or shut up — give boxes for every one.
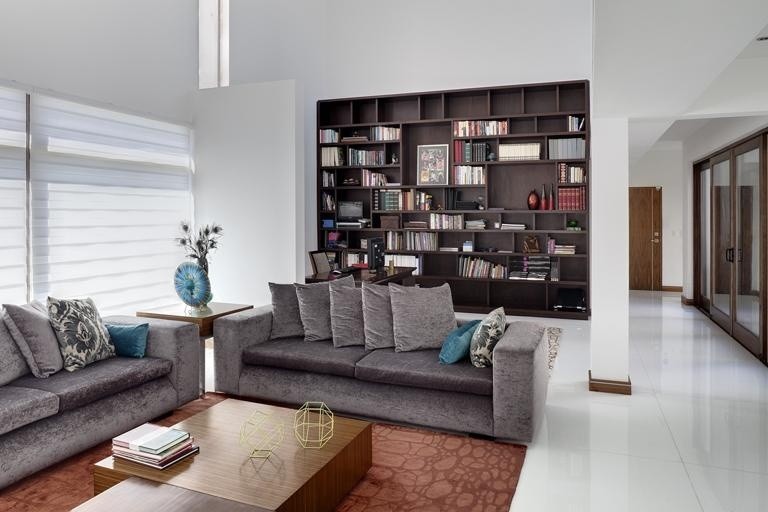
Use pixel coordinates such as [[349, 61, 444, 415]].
[[416, 143, 450, 185]]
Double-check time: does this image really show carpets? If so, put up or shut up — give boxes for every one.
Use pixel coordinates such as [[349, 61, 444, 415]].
[[0, 391, 528, 511], [547, 326, 561, 375]]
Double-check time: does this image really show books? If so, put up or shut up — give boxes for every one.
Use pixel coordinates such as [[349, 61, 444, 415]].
[[112, 422, 200, 471], [320, 115, 587, 281]]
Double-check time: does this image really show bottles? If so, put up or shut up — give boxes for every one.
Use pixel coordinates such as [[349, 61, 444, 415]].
[[392, 153, 398, 164], [541, 184, 547, 209], [549, 183, 554, 209]]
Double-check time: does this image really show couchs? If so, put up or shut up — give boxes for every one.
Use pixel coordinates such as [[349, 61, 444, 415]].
[[214, 307, 549, 447], [1, 314, 200, 488]]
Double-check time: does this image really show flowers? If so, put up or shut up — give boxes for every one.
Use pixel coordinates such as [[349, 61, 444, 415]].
[[178, 223, 223, 256]]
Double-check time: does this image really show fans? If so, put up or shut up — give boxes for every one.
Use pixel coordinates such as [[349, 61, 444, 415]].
[[174, 262, 212, 308]]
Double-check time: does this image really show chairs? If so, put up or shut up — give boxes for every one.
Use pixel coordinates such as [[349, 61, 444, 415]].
[[309, 251, 331, 274]]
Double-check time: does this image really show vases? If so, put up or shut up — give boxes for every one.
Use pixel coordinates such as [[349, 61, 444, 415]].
[[197, 257, 212, 312]]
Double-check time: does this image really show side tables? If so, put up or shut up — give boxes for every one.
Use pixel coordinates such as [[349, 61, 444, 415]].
[[136, 302, 253, 400]]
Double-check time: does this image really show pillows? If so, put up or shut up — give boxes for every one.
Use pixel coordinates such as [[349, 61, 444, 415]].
[[439, 319, 481, 365], [471, 306, 506, 368], [327, 281, 364, 348], [268, 281, 305, 339], [294, 274, 355, 341], [105, 321, 149, 357], [0, 299, 63, 378], [387, 281, 459, 354], [0, 319, 31, 387], [47, 296, 116, 371], [360, 280, 421, 352]]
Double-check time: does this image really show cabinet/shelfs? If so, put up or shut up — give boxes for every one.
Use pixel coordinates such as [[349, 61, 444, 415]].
[[305, 79, 591, 320]]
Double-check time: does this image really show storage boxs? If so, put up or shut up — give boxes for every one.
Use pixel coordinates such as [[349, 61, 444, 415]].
[[380, 215, 400, 229]]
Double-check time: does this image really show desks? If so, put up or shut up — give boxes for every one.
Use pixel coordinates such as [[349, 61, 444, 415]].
[[305, 266, 417, 286], [70, 475, 273, 511], [94, 397, 373, 511]]
